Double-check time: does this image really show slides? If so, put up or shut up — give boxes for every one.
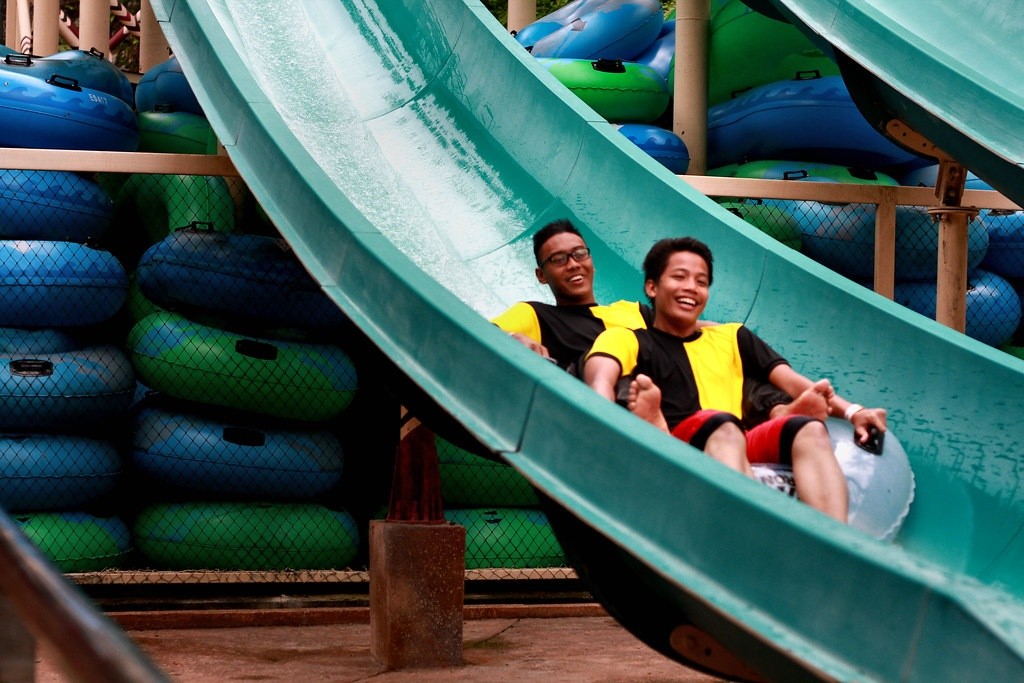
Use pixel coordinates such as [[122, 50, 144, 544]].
[[154, 0, 1024, 683]]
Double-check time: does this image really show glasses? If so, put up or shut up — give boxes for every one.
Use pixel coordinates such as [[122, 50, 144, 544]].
[[543, 247, 591, 267]]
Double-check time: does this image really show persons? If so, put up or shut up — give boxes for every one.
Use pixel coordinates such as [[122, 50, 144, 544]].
[[488, 216, 836, 435], [583, 237, 888, 526]]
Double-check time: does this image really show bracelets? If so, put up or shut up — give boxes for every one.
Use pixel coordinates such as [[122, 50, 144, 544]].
[[508, 332, 517, 336], [843, 403, 865, 424]]
